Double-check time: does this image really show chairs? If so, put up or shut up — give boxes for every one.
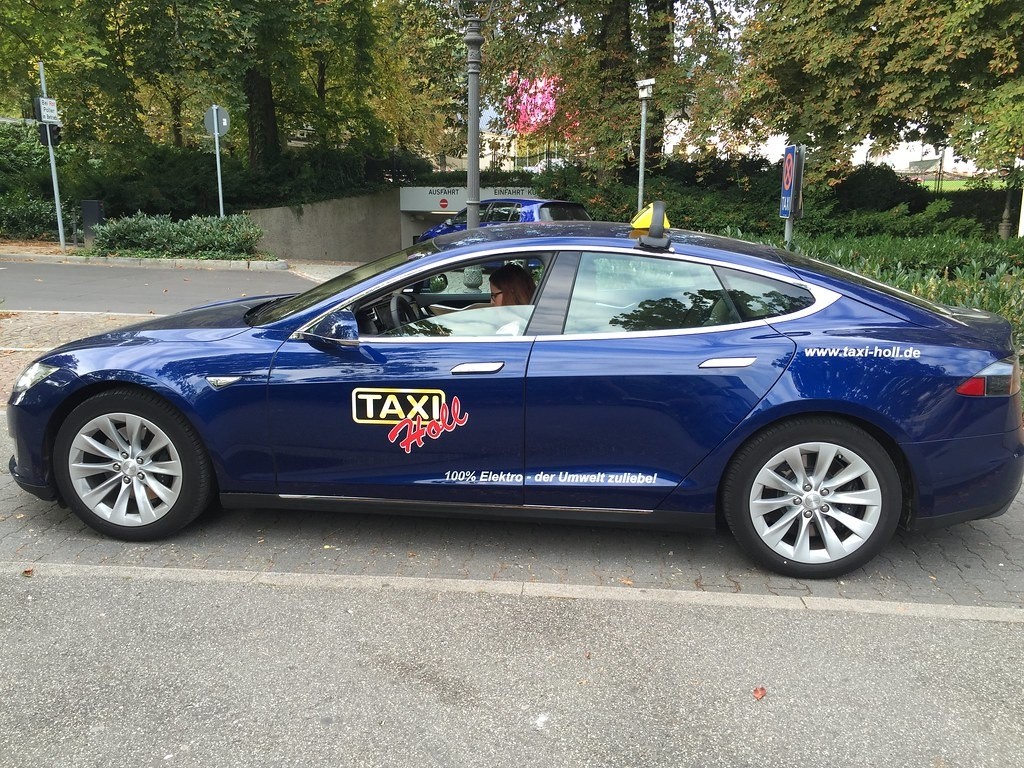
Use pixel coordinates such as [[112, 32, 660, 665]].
[[682, 295, 732, 326], [561, 260, 600, 334]]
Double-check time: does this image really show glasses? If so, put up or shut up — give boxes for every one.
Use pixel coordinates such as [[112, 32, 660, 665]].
[[491, 291, 503, 302]]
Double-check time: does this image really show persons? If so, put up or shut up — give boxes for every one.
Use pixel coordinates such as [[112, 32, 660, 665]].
[[424, 265, 537, 337]]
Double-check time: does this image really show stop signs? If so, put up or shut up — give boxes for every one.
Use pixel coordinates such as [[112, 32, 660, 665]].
[[437, 199, 450, 209]]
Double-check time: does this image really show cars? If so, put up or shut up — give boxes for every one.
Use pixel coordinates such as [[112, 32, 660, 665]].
[[415, 196, 593, 244], [4, 194, 1023, 579]]
